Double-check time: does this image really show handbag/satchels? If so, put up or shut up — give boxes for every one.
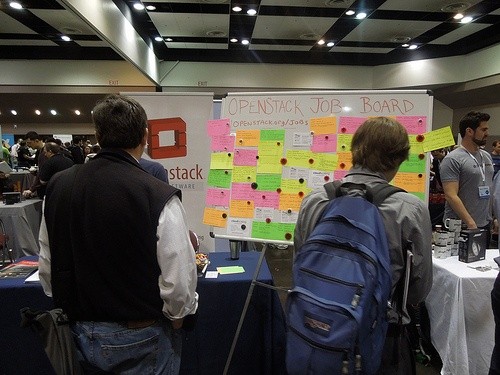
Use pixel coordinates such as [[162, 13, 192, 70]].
[[22, 309, 81, 375]]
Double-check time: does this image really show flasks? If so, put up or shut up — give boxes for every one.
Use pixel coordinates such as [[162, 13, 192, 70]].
[[229, 240, 242, 260]]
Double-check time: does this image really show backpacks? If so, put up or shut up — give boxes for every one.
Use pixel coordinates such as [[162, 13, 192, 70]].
[[285, 179, 407, 375]]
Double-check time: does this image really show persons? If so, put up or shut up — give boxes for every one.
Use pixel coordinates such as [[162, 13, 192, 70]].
[[0, 130, 168, 199], [38, 93, 198, 375], [293, 115, 433, 375], [429, 111, 500, 250]]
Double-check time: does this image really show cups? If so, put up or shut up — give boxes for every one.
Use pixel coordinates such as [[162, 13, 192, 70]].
[[434, 219, 463, 258]]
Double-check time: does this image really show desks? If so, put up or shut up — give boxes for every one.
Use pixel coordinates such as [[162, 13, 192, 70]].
[[182, 249, 287, 375], [423, 246, 500, 375], [0, 198, 42, 259], [0, 255, 55, 374]]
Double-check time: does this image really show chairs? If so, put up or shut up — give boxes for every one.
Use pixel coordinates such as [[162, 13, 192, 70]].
[[0, 219, 15, 267]]
